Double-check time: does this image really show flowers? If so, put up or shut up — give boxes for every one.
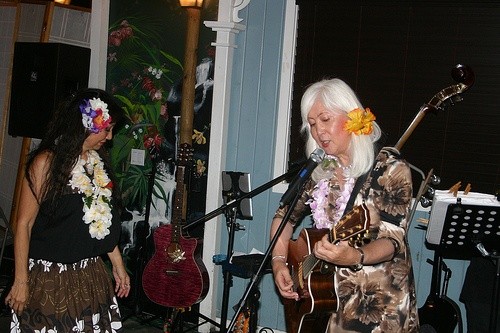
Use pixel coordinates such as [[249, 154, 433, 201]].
[[80, 97, 112, 134], [192, 126, 209, 145], [67, 149, 113, 240], [305, 156, 354, 229], [120, 66, 168, 158], [196, 159, 206, 176], [344, 108, 377, 137], [109, 19, 133, 47]]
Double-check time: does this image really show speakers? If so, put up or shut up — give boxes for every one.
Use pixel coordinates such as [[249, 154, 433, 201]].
[[8, 41, 91, 139]]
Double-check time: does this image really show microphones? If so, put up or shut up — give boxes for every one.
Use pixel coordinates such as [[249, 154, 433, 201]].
[[279, 147, 326, 208]]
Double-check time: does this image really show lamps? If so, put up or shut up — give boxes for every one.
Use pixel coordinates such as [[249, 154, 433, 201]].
[[178, 0, 205, 10]]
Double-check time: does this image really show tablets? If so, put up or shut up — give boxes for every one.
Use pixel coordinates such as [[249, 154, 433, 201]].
[[222, 171, 254, 220]]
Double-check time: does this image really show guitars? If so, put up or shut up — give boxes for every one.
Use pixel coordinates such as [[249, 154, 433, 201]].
[[285, 200, 371, 333], [142, 143, 210, 308]]
